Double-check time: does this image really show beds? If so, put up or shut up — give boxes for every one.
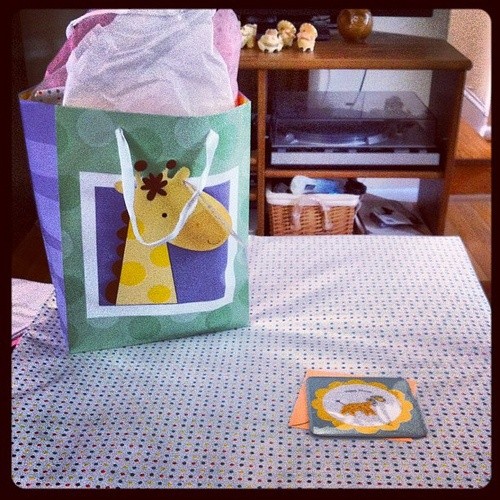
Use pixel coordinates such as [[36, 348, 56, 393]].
[[10, 232, 491, 489]]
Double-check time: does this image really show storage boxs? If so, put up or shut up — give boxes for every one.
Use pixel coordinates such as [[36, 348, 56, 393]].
[[263, 182, 366, 236]]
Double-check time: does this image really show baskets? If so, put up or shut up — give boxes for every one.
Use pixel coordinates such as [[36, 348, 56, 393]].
[[265, 182, 364, 234]]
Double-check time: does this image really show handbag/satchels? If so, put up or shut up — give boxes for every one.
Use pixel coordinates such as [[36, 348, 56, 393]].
[[12, 87, 254, 354]]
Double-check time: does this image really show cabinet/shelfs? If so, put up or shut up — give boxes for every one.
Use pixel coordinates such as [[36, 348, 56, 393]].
[[239, 25, 474, 234]]
